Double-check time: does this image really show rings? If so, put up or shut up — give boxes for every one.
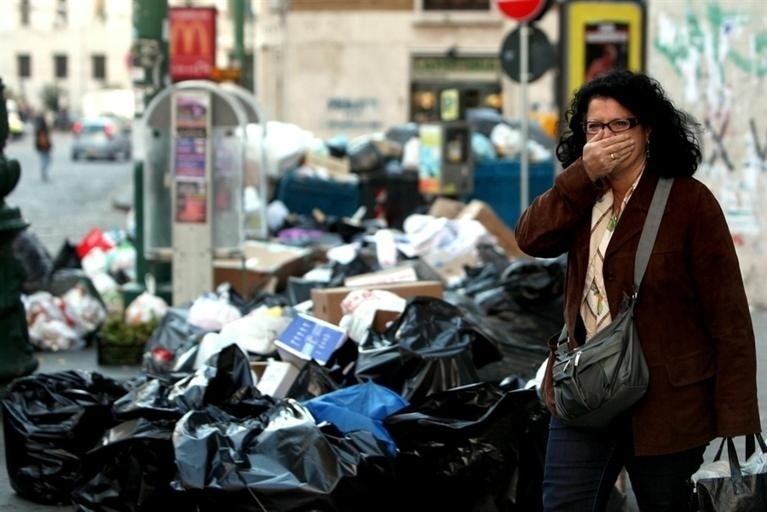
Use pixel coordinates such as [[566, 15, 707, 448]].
[[611, 153, 616, 160]]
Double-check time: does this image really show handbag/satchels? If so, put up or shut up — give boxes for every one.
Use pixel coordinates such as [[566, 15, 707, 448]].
[[553, 304, 650, 428], [688, 474, 767, 512]]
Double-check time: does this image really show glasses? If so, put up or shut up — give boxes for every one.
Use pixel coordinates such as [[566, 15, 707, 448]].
[[583, 116, 640, 134]]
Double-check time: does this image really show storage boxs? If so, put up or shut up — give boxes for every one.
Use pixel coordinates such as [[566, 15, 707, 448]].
[[213, 237, 314, 301], [309, 280, 442, 338], [429, 196, 525, 262], [278, 167, 363, 218]]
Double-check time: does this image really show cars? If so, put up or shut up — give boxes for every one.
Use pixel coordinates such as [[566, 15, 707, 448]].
[[71, 112, 131, 160]]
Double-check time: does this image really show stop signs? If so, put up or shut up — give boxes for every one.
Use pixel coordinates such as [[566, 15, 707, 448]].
[[495, 0, 553, 24]]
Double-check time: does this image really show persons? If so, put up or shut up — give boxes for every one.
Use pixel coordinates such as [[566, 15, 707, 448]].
[[515, 71, 756, 511], [33, 104, 54, 182], [587, 44, 620, 82]]
